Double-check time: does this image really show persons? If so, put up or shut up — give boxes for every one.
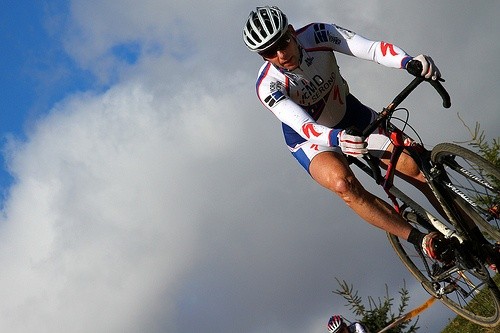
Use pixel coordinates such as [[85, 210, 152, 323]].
[[325, 315, 364, 333], [243, 5, 500, 271]]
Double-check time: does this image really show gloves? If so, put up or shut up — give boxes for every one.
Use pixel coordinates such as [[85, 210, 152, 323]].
[[337, 129, 368, 157], [408, 54, 441, 80]]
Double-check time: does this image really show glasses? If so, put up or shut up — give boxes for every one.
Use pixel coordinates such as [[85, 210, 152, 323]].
[[260, 31, 293, 57]]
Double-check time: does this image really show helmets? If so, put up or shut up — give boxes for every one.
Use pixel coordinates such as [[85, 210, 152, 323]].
[[241, 6, 288, 53], [326, 315, 344, 333]]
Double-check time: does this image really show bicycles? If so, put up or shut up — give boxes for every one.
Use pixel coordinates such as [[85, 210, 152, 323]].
[[346, 59, 500, 328]]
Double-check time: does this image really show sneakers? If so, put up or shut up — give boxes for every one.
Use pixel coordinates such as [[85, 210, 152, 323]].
[[422, 231, 451, 266]]
